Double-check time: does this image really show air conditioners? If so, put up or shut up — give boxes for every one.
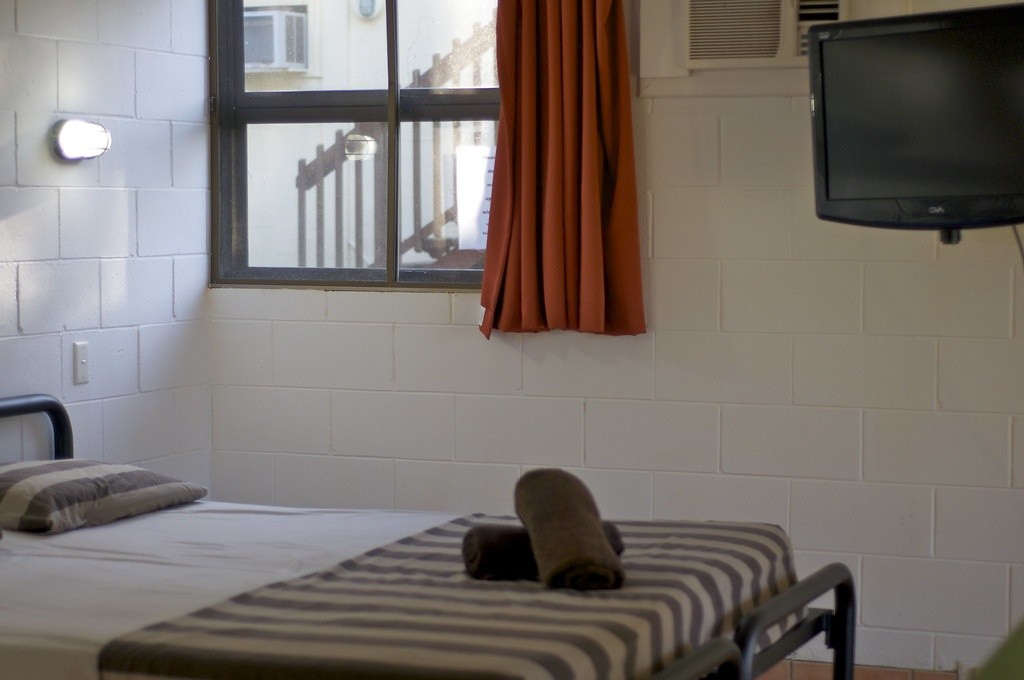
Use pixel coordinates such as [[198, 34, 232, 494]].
[[243, 12, 310, 75]]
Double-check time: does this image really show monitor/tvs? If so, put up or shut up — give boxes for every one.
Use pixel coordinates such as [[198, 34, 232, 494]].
[[807, 0, 1024, 231]]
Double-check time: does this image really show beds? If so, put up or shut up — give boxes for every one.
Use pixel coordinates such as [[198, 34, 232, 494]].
[[0, 394, 866, 680]]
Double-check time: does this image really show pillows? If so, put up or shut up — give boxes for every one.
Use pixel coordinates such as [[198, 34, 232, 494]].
[[0, 455, 208, 536]]
